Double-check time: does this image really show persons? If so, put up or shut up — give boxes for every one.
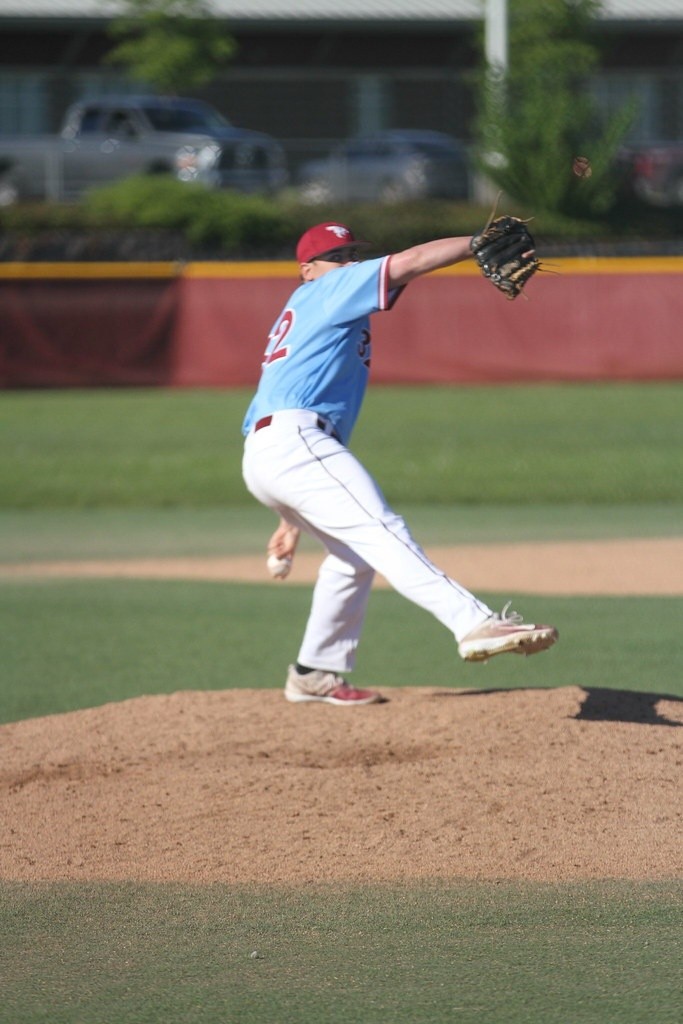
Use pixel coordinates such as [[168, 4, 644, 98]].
[[239, 222, 558, 706]]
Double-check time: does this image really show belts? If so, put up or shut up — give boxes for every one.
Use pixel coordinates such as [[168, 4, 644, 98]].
[[255, 415, 340, 442]]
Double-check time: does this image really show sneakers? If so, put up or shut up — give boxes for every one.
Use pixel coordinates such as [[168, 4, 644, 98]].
[[458, 601, 560, 663], [284, 665, 381, 706]]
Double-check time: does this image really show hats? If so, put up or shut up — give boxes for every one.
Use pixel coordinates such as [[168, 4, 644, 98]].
[[296, 222, 373, 265]]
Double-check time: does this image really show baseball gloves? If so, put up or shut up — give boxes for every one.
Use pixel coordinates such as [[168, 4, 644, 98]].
[[468, 214, 543, 302]]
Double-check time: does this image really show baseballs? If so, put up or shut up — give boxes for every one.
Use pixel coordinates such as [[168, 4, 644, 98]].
[[266, 552, 294, 579]]
